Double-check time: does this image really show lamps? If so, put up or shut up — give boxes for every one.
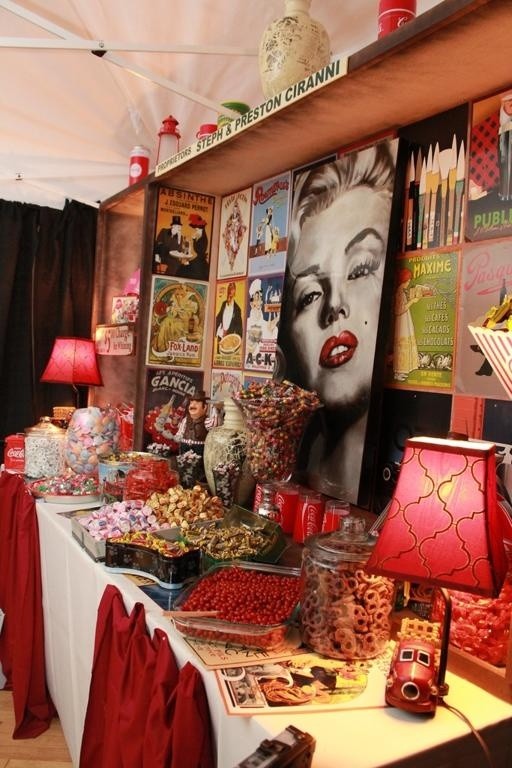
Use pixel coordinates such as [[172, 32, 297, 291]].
[[363, 436, 508, 699], [40, 335, 106, 407]]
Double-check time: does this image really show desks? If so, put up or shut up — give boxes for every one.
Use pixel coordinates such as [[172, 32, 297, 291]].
[[0, 462, 512, 768]]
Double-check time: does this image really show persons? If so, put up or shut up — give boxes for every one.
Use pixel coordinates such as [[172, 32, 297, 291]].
[[395, 261, 424, 380]]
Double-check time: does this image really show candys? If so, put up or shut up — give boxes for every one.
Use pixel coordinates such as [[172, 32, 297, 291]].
[[170, 562, 305, 650], [32, 405, 124, 504], [70, 484, 291, 590], [229, 380, 320, 487], [302, 518, 394, 658], [431, 580, 512, 668]]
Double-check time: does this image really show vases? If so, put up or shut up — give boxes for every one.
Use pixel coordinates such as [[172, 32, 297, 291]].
[[202, 395, 259, 507]]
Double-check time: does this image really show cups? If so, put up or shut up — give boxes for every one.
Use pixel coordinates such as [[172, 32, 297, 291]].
[[128, 144, 150, 192], [252, 480, 351, 545]]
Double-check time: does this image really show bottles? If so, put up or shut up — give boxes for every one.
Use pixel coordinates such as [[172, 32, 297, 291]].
[[188, 311, 195, 334], [25, 415, 68, 480], [301, 516, 396, 661], [178, 235, 190, 255]]
